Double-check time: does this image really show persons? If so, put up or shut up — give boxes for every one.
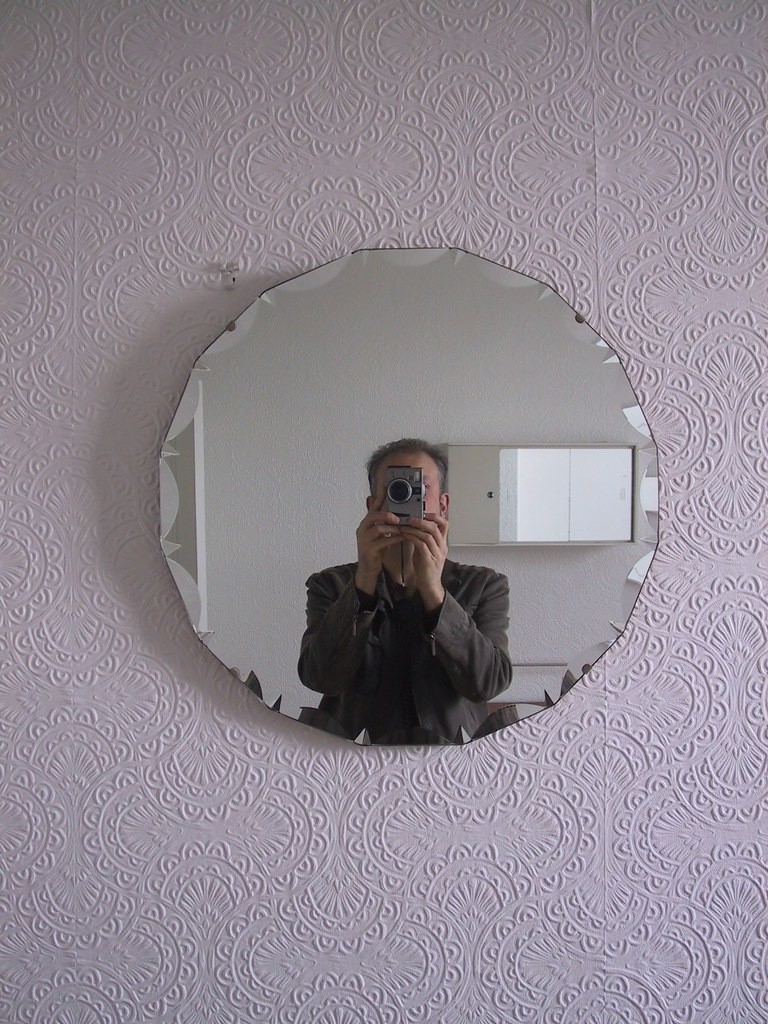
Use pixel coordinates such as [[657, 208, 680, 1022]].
[[296, 438, 512, 746]]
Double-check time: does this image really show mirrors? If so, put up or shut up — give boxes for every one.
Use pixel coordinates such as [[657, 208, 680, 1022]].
[[159, 249, 661, 746]]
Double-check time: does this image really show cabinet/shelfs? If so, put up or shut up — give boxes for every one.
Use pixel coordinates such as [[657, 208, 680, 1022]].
[[437, 442, 638, 549]]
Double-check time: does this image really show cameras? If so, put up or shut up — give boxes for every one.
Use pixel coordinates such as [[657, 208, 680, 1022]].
[[385, 465, 426, 529]]
